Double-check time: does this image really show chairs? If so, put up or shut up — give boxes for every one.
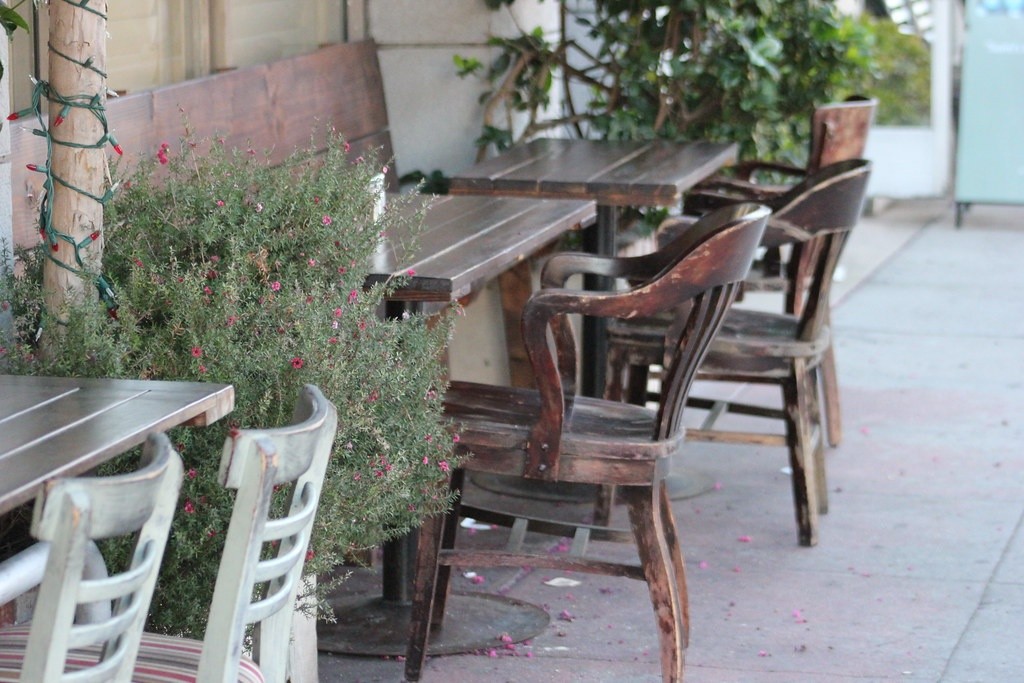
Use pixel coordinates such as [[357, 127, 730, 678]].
[[378, 199, 770, 681], [681, 100, 879, 451], [0, 382, 339, 682], [1, 428, 185, 681], [602, 159, 876, 552]]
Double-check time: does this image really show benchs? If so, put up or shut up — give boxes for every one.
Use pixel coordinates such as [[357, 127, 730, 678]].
[[9, 40, 447, 288]]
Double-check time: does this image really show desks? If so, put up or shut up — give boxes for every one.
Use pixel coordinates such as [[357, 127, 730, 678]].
[[460, 131, 742, 406], [251, 181, 606, 652], [2, 370, 234, 517]]
